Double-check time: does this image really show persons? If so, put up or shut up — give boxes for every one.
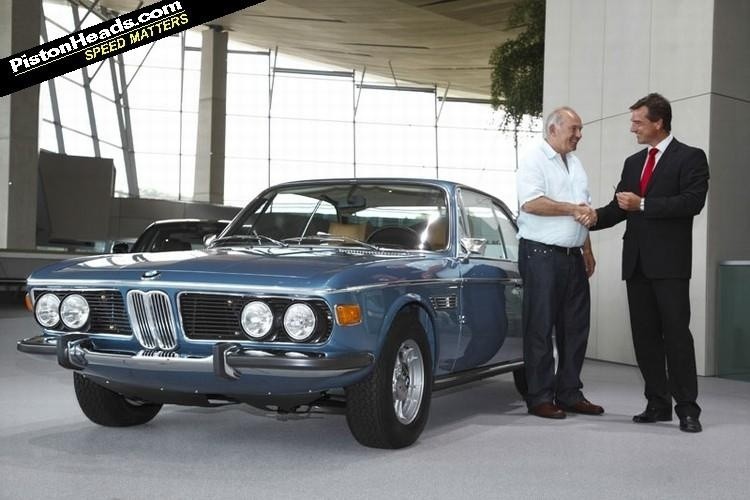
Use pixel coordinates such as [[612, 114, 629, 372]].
[[575, 93, 710, 432], [515, 107, 605, 420]]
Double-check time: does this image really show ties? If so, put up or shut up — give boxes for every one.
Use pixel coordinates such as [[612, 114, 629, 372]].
[[638, 148, 659, 200]]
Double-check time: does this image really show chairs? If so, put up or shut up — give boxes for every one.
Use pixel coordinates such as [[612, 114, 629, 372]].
[[427, 215, 449, 251], [327, 220, 371, 246]]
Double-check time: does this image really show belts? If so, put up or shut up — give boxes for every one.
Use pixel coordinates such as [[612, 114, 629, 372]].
[[520, 238, 584, 256]]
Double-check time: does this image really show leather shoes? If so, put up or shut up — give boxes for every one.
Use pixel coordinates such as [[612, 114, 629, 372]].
[[633, 402, 703, 433], [528, 398, 605, 419]]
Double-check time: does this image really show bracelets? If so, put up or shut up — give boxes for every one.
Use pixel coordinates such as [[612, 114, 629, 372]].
[[639, 196, 646, 213]]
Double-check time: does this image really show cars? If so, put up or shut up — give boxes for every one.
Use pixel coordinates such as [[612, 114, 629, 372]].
[[16, 177, 526, 450], [109, 218, 253, 255]]
